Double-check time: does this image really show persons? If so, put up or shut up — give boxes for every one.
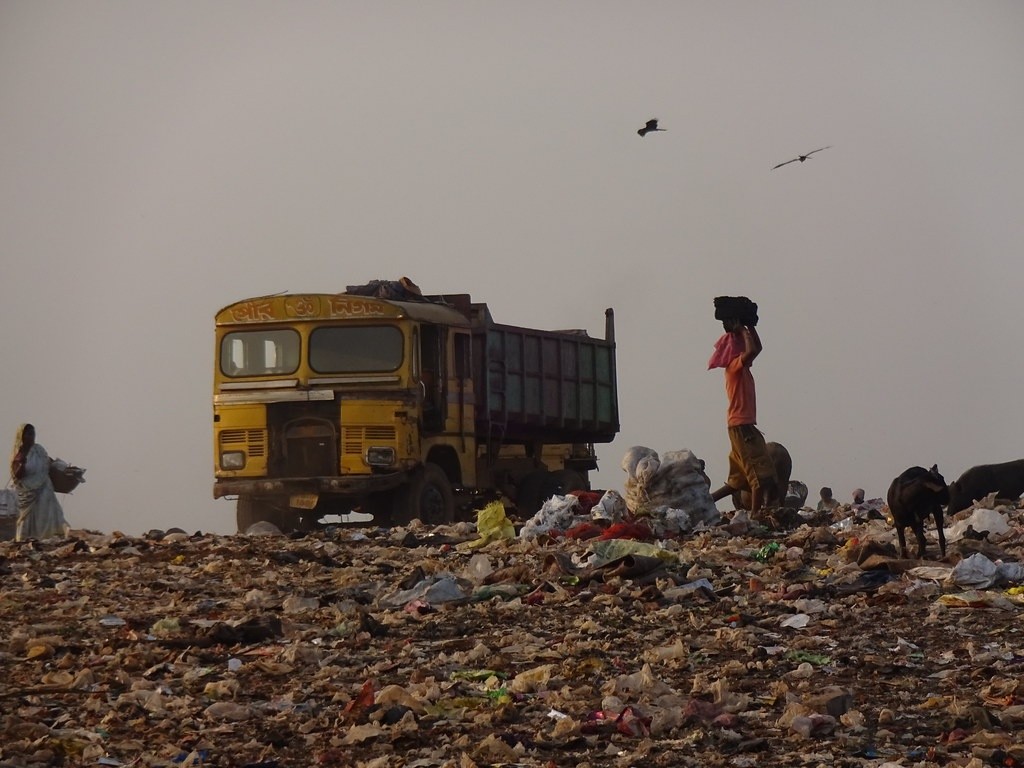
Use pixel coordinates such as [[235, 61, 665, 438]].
[[817, 487, 840, 511], [709, 317, 775, 521], [850, 489, 866, 506], [10, 423, 72, 541]]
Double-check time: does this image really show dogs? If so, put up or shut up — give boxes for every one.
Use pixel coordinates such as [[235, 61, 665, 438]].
[[947, 459, 1024, 515], [887, 464, 951, 561]]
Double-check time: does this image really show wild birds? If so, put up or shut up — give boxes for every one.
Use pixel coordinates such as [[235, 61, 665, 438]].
[[637, 119, 667, 137], [769, 145, 829, 170]]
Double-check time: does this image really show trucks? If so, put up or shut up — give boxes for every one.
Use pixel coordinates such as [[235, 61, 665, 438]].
[[211, 274, 622, 535]]
